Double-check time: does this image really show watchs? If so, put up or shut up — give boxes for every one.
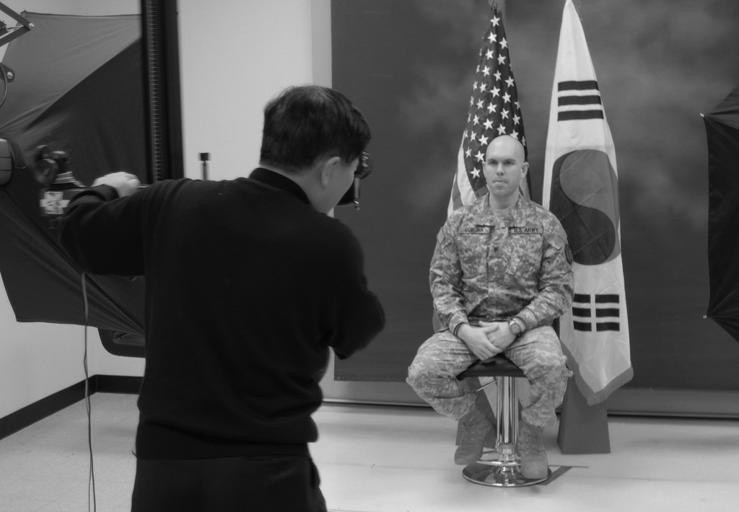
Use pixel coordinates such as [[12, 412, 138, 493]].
[[507, 317, 522, 338]]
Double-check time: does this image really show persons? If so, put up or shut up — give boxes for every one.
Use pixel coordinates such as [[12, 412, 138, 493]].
[[405, 135, 575, 480], [59, 86, 385, 512]]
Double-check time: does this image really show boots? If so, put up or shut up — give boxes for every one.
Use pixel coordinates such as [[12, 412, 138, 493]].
[[515, 421, 550, 480], [452, 411, 497, 465]]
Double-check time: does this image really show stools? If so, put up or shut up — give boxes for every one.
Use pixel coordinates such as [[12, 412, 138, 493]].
[[460, 355, 552, 487]]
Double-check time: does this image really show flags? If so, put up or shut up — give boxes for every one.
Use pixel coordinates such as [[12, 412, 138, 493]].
[[447, 1, 533, 218], [542, 1, 635, 405]]
